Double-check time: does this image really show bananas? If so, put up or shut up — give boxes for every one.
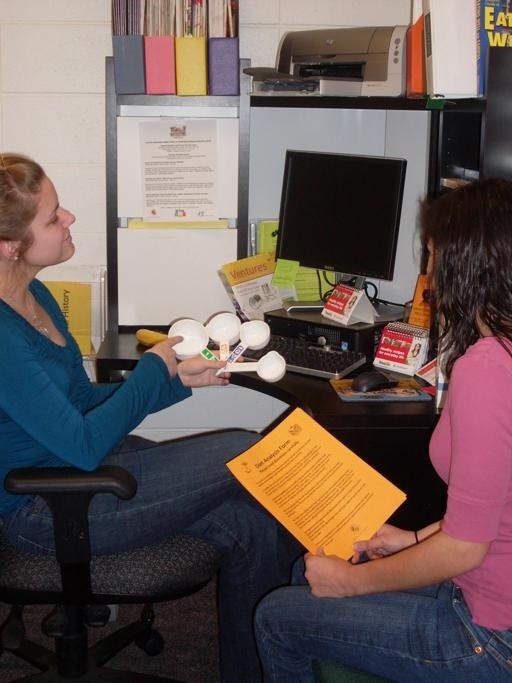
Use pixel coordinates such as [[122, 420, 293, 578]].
[[136, 329, 168, 347]]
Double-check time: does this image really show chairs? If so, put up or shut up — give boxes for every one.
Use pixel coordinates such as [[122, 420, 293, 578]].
[[316, 657, 390, 683], [0, 359, 215, 683]]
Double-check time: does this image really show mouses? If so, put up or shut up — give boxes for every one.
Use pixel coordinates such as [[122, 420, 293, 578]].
[[351, 370, 399, 392]]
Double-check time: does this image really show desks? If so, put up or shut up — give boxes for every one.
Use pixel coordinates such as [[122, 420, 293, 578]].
[[94, 328, 439, 587]]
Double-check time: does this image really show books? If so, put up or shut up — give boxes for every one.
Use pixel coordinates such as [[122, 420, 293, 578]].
[[111, 0, 238, 39], [218, 219, 336, 322]]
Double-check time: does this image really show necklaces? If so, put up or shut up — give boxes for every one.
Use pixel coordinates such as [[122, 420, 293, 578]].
[[15, 294, 50, 340]]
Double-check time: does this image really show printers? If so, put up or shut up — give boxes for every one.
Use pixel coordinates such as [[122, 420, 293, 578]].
[[243, 24, 411, 97]]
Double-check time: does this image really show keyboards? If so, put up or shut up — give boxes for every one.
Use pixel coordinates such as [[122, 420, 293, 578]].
[[229, 334, 368, 381]]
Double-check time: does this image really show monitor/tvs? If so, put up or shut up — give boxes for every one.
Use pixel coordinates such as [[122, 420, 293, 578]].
[[273, 149, 407, 312]]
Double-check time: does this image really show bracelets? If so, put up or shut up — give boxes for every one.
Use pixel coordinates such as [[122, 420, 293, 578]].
[[415, 530, 420, 544]]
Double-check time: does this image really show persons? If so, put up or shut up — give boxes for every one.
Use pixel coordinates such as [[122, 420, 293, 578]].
[[1, 152, 302, 683], [259, 168, 512, 683], [348, 295, 359, 308], [408, 343, 422, 367]]
